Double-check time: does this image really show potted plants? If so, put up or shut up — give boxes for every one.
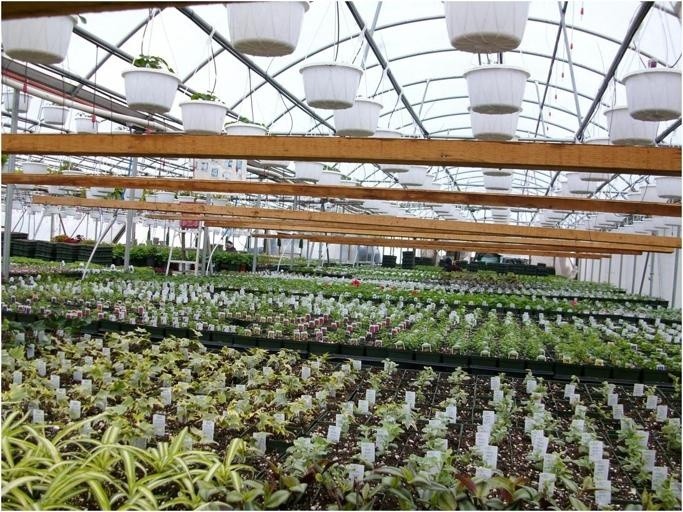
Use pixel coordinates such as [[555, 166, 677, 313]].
[[1, 1, 682, 511]]
